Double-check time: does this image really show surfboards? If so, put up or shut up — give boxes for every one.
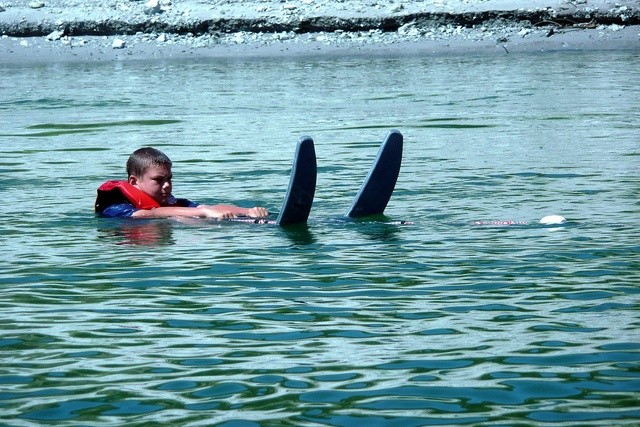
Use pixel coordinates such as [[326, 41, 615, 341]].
[[275, 135, 317, 225], [345, 129, 403, 216]]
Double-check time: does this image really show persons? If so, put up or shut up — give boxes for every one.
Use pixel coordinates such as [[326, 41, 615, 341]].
[[93, 144, 270, 223]]
